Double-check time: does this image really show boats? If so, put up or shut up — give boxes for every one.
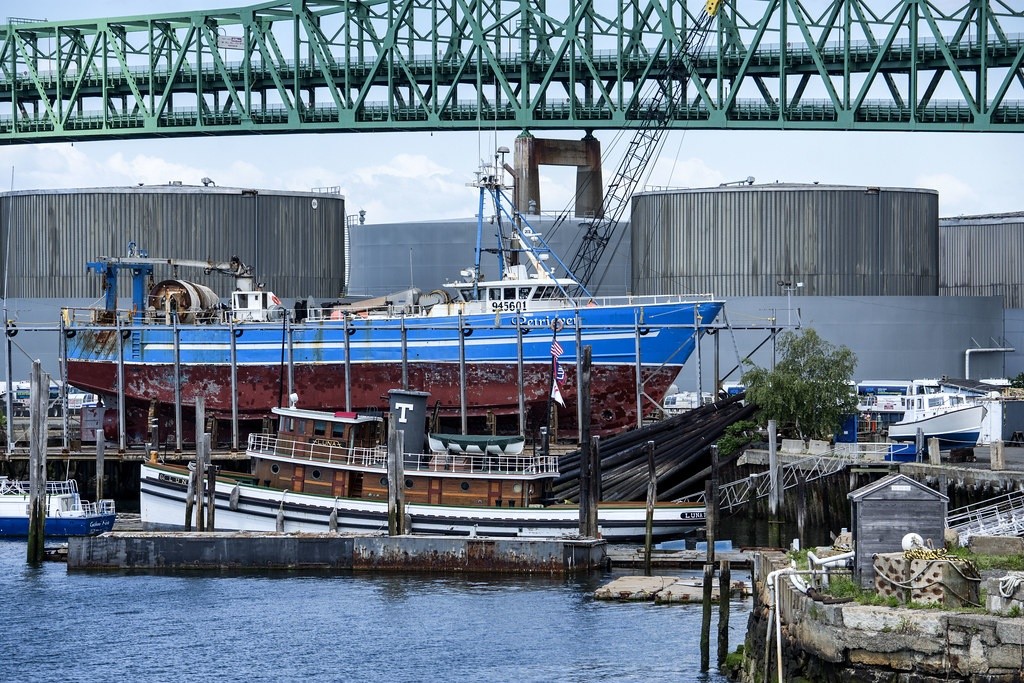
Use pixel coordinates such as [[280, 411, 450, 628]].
[[0, 142, 1024, 543]]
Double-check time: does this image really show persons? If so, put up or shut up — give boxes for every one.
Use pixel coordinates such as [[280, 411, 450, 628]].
[[294, 298, 302, 324]]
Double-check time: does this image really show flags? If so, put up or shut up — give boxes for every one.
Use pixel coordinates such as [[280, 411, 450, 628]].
[[549, 339, 563, 358], [551, 379, 566, 409], [553, 358, 567, 389]]
